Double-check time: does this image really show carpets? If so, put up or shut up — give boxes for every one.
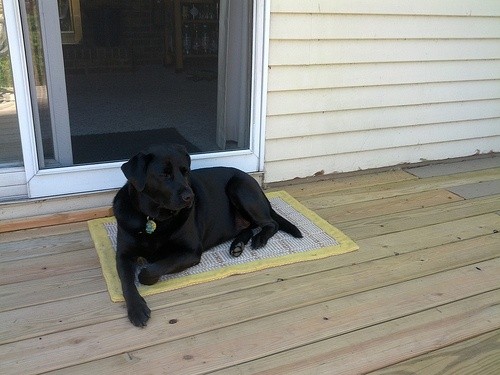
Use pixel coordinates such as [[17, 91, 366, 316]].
[[87, 190, 360, 302]]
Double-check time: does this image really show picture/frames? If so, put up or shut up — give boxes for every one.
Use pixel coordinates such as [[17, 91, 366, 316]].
[[57, 0, 82, 44]]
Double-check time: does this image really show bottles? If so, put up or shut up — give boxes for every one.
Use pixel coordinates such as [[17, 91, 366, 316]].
[[182, 2, 219, 56]]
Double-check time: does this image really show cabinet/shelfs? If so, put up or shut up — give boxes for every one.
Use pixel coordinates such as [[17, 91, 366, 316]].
[[165, 0, 220, 72]]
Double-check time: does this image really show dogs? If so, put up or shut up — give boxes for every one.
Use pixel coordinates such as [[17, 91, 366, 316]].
[[112, 142, 303, 327]]
